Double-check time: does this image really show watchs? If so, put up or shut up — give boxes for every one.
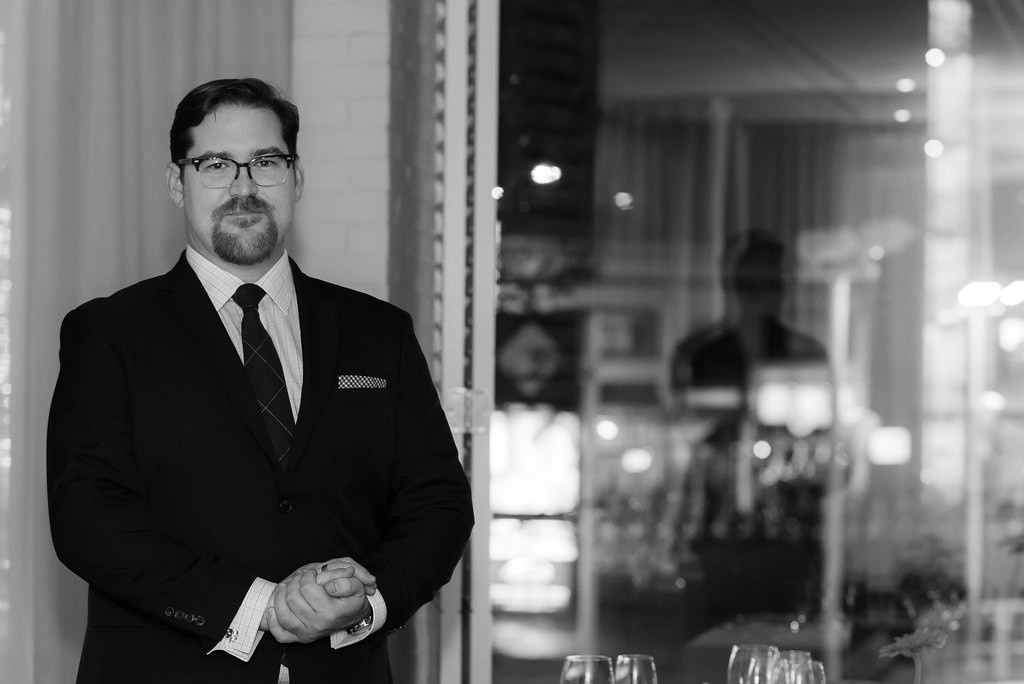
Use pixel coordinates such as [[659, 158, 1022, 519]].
[[347, 607, 378, 640]]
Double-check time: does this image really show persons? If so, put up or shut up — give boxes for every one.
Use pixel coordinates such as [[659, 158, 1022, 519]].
[[670, 223, 857, 656], [45, 76, 475, 684]]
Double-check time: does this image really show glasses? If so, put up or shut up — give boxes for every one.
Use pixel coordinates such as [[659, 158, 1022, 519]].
[[178, 153, 297, 189]]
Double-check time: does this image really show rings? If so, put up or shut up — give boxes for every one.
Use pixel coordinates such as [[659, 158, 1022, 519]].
[[321, 563, 329, 572]]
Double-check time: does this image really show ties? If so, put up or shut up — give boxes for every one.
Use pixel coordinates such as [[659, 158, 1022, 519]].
[[231, 283, 296, 475]]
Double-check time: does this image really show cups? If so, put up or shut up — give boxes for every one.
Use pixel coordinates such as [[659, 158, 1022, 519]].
[[726, 644, 780, 684], [766, 650, 811, 684], [802, 662, 825, 684], [615, 654, 656, 684], [560, 656, 614, 684]]
